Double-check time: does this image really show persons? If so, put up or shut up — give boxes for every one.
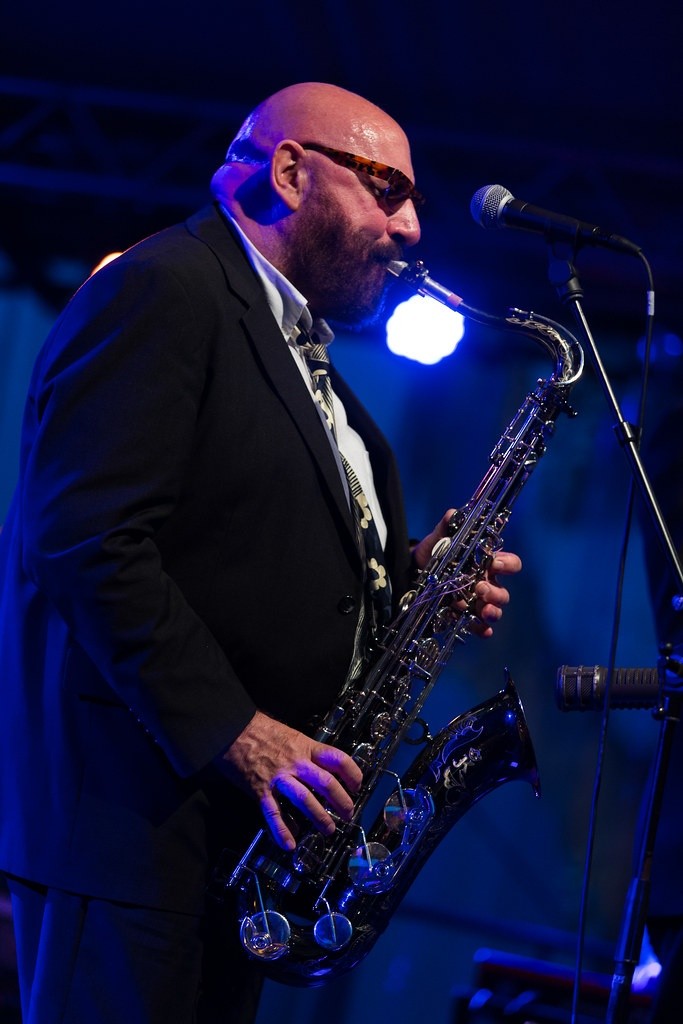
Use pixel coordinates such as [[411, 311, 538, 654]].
[[2, 77, 522, 1024]]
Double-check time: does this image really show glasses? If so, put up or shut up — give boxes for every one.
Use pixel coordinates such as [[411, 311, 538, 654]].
[[298, 142, 426, 216]]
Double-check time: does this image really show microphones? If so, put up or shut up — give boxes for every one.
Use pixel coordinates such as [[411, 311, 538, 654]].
[[469, 185, 642, 257]]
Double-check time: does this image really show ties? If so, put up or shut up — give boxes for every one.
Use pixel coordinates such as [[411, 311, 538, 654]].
[[288, 317, 393, 637]]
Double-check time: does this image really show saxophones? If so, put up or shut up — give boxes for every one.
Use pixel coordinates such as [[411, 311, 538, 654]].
[[223, 256, 588, 975]]
